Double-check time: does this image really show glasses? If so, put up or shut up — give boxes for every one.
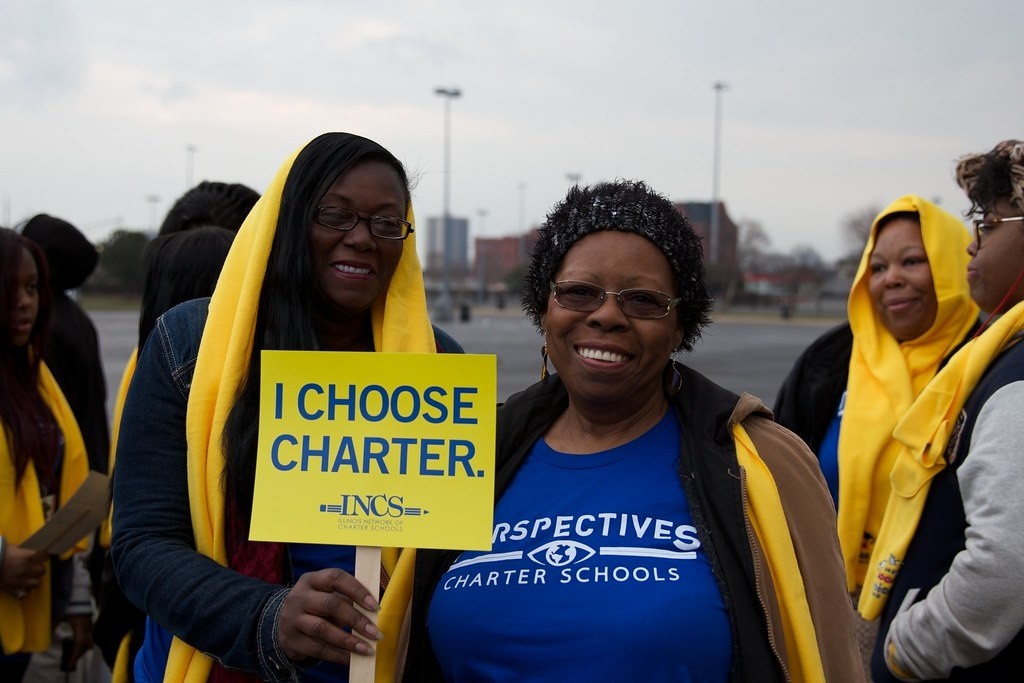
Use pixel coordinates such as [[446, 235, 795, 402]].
[[549, 279, 681, 319], [312, 205, 414, 241], [972, 216, 1024, 249]]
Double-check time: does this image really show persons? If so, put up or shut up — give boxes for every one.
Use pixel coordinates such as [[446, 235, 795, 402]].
[[850, 139, 1024, 682], [107, 130, 467, 683], [21, 214, 109, 683], [769, 189, 991, 610], [0, 226, 88, 683], [375, 178, 866, 683]]
